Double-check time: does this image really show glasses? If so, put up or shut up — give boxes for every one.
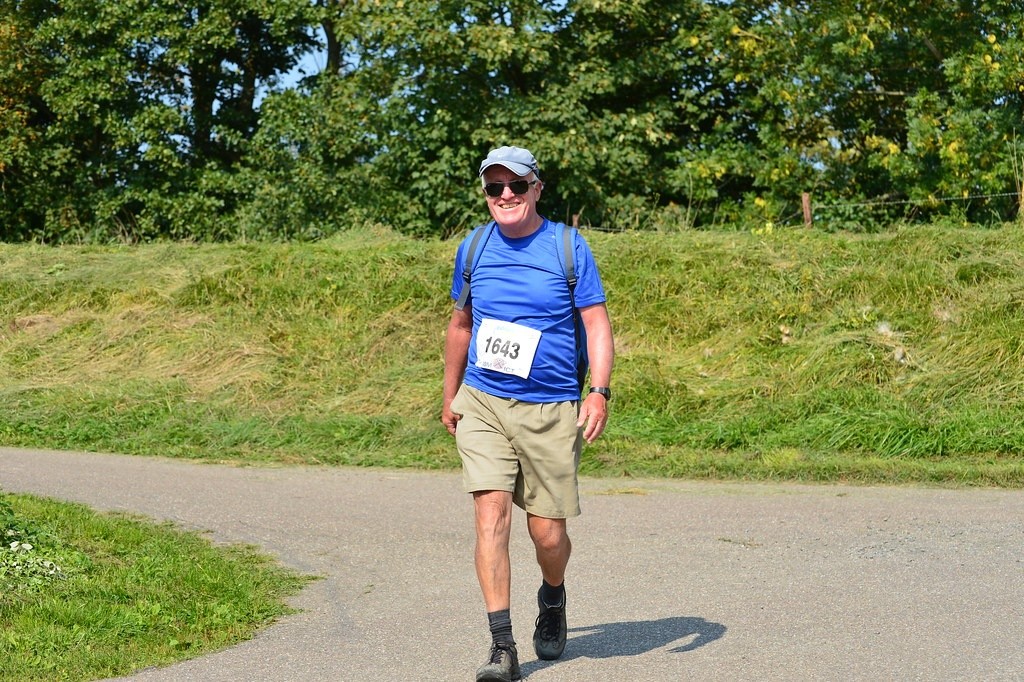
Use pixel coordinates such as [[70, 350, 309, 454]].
[[483, 179, 538, 197]]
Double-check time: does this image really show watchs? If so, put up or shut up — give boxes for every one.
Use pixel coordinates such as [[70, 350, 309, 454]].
[[588, 386, 613, 401]]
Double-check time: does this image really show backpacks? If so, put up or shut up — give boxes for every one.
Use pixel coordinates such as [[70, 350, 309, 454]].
[[461, 219, 590, 392]]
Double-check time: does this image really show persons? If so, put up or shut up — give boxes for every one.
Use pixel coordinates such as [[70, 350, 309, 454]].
[[440, 144, 614, 682]]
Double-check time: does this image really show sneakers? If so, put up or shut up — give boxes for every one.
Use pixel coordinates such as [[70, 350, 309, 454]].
[[475, 642, 521, 682], [533, 586, 567, 660]]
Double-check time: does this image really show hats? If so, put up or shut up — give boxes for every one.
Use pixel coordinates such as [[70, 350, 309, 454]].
[[478, 146, 540, 176]]
